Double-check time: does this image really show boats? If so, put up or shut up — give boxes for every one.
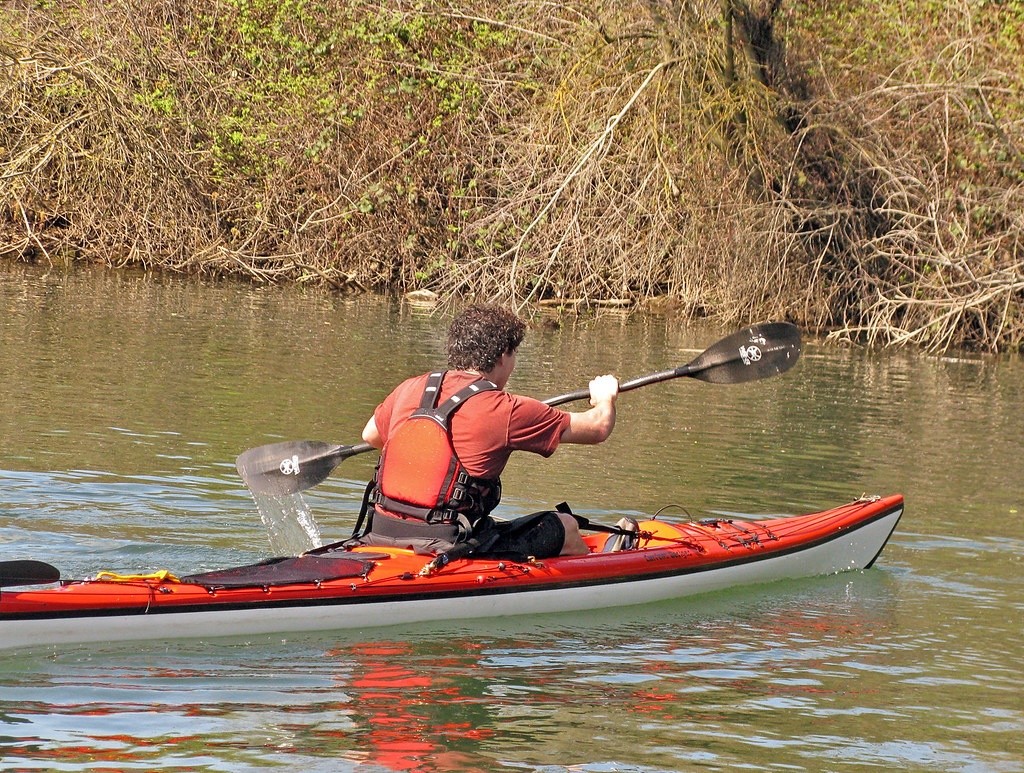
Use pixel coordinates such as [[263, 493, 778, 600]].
[[1, 489, 907, 650]]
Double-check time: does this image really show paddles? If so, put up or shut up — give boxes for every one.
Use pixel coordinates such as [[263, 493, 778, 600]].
[[237, 320, 805, 497]]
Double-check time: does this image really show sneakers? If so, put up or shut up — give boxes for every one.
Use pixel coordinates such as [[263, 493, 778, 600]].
[[602, 516, 640, 553]]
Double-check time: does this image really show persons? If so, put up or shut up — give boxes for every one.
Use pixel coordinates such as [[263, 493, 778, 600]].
[[362, 305, 620, 558]]
